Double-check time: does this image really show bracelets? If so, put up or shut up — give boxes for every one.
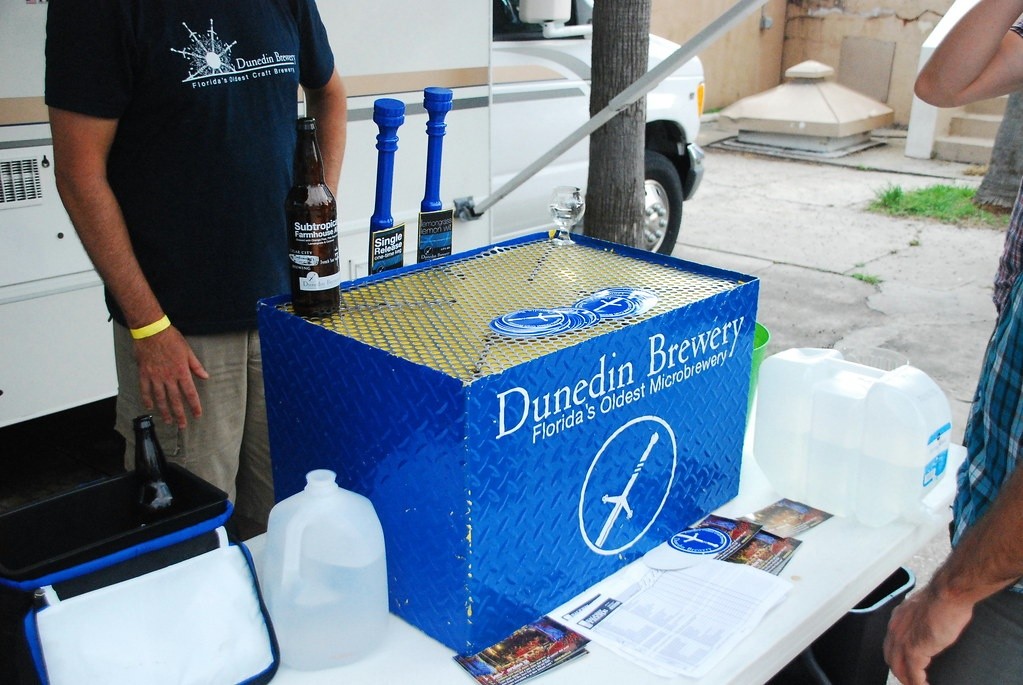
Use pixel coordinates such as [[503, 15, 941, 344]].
[[130, 312, 170, 340]]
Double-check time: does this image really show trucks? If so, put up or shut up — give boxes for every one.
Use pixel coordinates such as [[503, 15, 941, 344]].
[[2, 1, 707, 438]]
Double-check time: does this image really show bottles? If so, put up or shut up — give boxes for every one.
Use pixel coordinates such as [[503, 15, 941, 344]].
[[261, 469, 389, 672], [285, 117, 340, 319], [132, 415, 182, 528]]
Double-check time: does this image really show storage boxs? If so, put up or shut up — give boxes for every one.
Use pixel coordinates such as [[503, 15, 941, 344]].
[[0, 411, 283, 685], [255, 231, 760, 658]]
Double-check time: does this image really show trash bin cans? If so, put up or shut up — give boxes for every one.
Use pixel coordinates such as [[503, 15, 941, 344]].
[[811, 564, 916, 685]]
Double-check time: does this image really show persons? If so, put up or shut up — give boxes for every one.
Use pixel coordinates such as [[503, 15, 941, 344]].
[[44, 0, 347, 576], [885, 0, 1023, 685]]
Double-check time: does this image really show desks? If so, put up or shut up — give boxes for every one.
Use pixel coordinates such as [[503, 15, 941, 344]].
[[242, 438, 970, 685]]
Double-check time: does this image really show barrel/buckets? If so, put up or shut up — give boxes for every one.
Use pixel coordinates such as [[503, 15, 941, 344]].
[[745, 321, 770, 434]]
